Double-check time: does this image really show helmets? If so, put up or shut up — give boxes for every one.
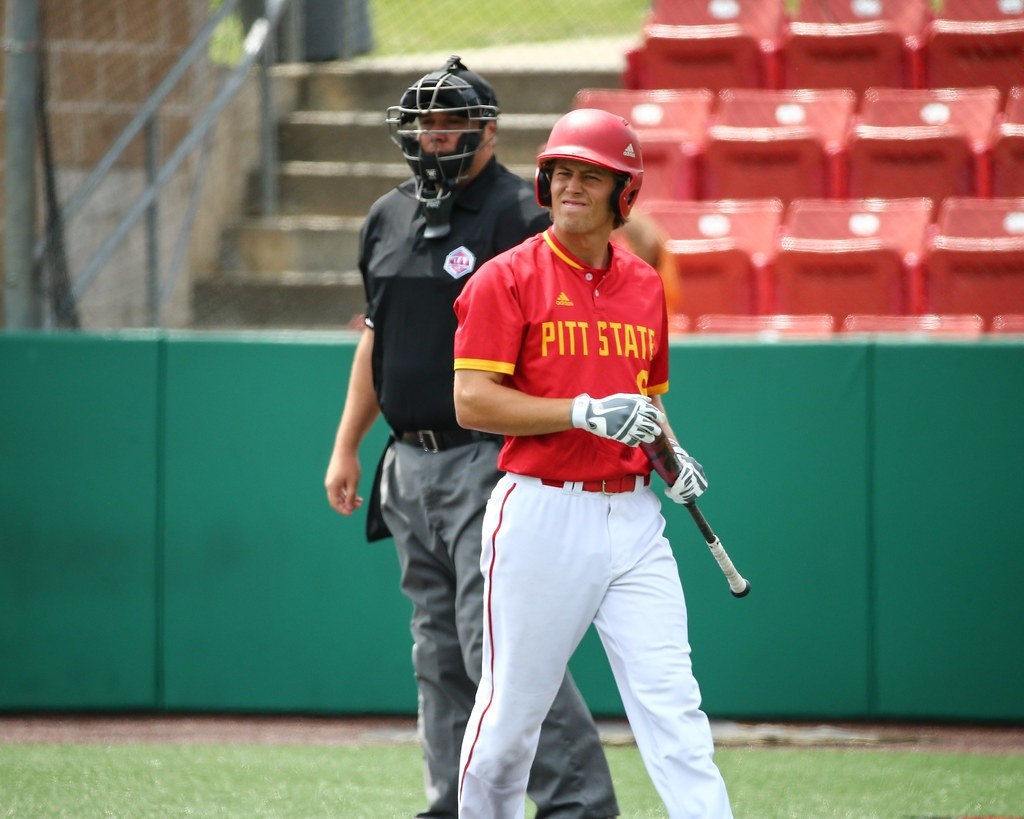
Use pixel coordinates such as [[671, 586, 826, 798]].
[[534, 109, 644, 223], [386, 55, 500, 208]]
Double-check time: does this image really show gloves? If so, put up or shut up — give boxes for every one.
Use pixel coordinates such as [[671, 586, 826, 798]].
[[664, 437, 708, 504], [572, 392, 666, 448]]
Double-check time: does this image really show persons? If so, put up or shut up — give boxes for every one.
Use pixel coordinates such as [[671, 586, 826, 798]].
[[324, 54, 620, 819], [450, 109, 735, 819]]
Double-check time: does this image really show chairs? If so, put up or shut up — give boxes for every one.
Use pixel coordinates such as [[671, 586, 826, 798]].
[[560, 0, 1024, 340]]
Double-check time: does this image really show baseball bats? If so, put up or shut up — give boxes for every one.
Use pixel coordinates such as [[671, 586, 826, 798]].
[[638, 419, 752, 598]]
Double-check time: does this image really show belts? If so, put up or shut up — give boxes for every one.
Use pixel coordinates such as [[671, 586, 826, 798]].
[[542, 474, 650, 496], [395, 428, 498, 454]]
